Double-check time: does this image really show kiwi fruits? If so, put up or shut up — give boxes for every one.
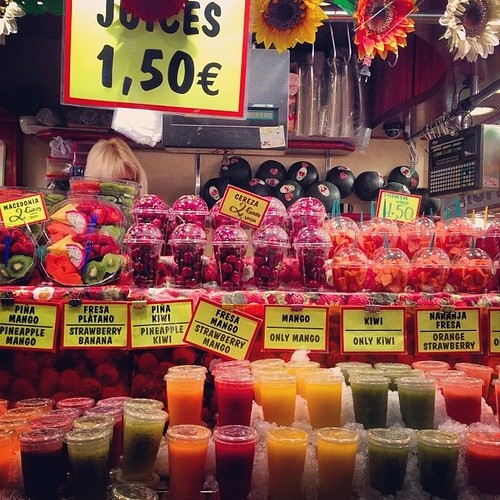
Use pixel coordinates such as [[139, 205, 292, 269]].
[[83, 183, 136, 283], [0, 255, 35, 283], [24, 193, 65, 239]]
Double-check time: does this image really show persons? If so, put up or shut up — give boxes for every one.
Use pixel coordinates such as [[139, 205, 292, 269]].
[[84, 137, 148, 195]]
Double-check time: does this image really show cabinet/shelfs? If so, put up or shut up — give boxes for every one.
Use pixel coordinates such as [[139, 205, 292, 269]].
[[365, 0, 500, 139]]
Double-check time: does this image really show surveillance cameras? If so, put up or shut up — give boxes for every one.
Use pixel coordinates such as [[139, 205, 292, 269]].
[[383, 123, 402, 139]]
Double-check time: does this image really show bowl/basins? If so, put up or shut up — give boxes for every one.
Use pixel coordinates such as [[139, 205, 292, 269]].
[[45, 157, 73, 176], [0, 175, 142, 287]]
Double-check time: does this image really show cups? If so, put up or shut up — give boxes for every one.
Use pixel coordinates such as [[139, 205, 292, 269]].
[[301, 53, 355, 138], [1, 359, 500, 500], [123, 194, 500, 295]]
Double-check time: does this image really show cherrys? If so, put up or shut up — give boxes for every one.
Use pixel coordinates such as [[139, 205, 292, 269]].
[[127, 196, 326, 288]]
